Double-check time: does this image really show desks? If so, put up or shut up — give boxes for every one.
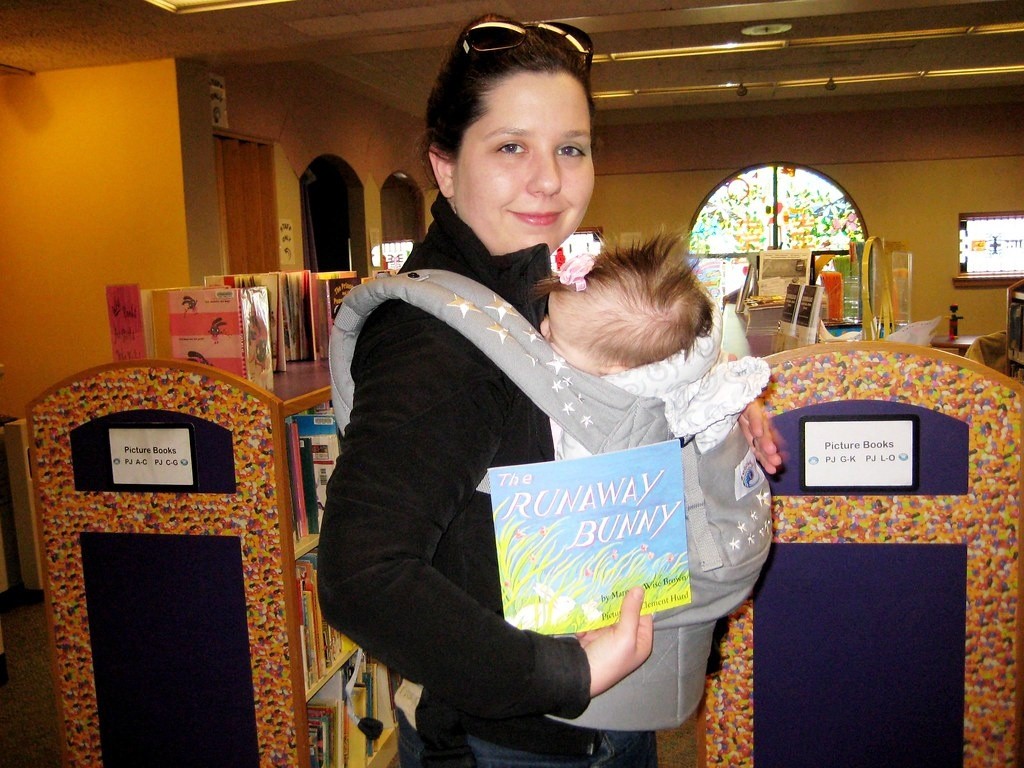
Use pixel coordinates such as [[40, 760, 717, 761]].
[[930, 336, 976, 357]]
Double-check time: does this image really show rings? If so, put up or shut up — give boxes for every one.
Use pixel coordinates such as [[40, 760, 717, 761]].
[[753, 438, 755, 447]]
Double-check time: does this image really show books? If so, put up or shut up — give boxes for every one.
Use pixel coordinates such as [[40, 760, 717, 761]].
[[139, 270, 358, 392], [780, 282, 825, 346], [285, 407, 401, 768], [487, 439, 691, 635]]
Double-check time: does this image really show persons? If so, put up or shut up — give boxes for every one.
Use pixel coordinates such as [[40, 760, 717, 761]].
[[317, 13, 782, 768], [535, 230, 773, 627]]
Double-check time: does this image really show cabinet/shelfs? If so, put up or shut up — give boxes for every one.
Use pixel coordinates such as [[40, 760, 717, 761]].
[[24, 357, 398, 768], [1005, 280, 1024, 386]]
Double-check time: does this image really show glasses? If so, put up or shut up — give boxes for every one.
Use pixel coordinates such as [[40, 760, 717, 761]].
[[452, 22, 593, 78]]
